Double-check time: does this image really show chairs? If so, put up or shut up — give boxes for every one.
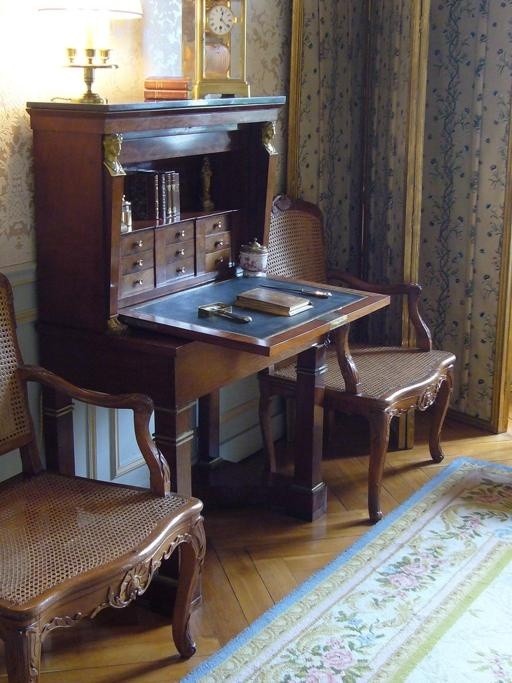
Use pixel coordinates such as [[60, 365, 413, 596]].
[[0, 272, 211, 680], [250, 189, 461, 524]]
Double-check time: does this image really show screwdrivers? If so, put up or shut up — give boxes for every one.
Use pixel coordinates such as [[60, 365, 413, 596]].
[[260, 284, 333, 298]]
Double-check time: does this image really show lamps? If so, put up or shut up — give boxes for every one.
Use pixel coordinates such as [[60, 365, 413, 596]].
[[36, 0, 147, 101]]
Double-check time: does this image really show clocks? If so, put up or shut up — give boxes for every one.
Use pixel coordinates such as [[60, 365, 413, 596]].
[[179, 1, 252, 96]]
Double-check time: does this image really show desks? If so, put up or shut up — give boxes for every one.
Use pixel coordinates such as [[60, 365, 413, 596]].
[[23, 275, 391, 617]]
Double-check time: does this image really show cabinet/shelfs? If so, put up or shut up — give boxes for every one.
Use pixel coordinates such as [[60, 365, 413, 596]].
[[25, 94, 290, 335]]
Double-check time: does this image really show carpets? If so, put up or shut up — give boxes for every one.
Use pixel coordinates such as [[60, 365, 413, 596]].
[[178, 454, 511, 682]]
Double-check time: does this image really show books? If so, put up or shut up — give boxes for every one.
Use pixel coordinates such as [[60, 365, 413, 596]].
[[124, 170, 180, 226], [235, 287, 314, 317]]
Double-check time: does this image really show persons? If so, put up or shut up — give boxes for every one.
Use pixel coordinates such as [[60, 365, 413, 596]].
[[103, 133, 128, 175], [262, 122, 280, 157]]
